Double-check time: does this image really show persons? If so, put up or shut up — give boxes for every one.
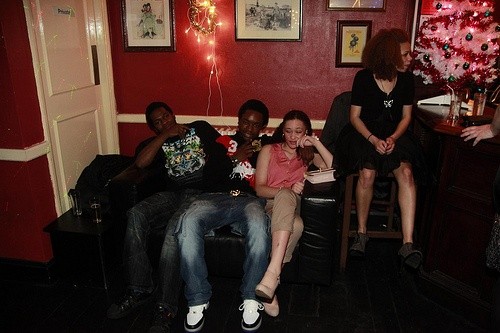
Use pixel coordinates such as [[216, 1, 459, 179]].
[[174, 97, 295, 333], [459, 81, 500, 281], [253, 109, 337, 318], [107, 101, 222, 332], [346, 26, 427, 269]]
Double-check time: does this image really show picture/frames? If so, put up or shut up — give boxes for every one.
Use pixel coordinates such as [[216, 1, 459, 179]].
[[234, 0, 303, 42], [326, 0, 387, 12], [120, 0, 176, 52], [336, 20, 372, 68]]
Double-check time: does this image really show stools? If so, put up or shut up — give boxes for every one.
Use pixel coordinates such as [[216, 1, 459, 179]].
[[319, 91, 402, 275]]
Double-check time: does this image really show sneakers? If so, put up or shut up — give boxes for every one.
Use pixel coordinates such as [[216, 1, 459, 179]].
[[238, 299, 264, 331], [106, 291, 148, 318], [183, 300, 210, 332], [149, 305, 173, 333]]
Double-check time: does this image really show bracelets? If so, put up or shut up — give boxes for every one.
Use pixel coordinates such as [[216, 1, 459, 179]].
[[367, 134, 373, 141]]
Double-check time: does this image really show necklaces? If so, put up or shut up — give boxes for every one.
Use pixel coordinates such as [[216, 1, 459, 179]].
[[379, 77, 395, 94]]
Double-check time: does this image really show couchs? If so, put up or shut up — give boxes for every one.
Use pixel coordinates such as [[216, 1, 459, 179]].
[[104, 135, 345, 286]]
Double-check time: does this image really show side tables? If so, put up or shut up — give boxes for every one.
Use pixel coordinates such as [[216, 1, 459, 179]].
[[42, 208, 125, 296]]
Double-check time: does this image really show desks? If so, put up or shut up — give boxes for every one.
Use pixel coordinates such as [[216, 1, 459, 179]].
[[410, 105, 500, 333]]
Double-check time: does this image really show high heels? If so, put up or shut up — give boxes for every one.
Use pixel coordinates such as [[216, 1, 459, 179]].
[[259, 293, 280, 317], [256, 270, 280, 302], [350, 230, 368, 255], [397, 243, 422, 278]]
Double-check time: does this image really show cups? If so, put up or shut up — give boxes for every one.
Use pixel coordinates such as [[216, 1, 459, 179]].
[[448, 93, 461, 120], [89, 196, 102, 223], [70, 190, 82, 215], [473, 92, 487, 116]]
